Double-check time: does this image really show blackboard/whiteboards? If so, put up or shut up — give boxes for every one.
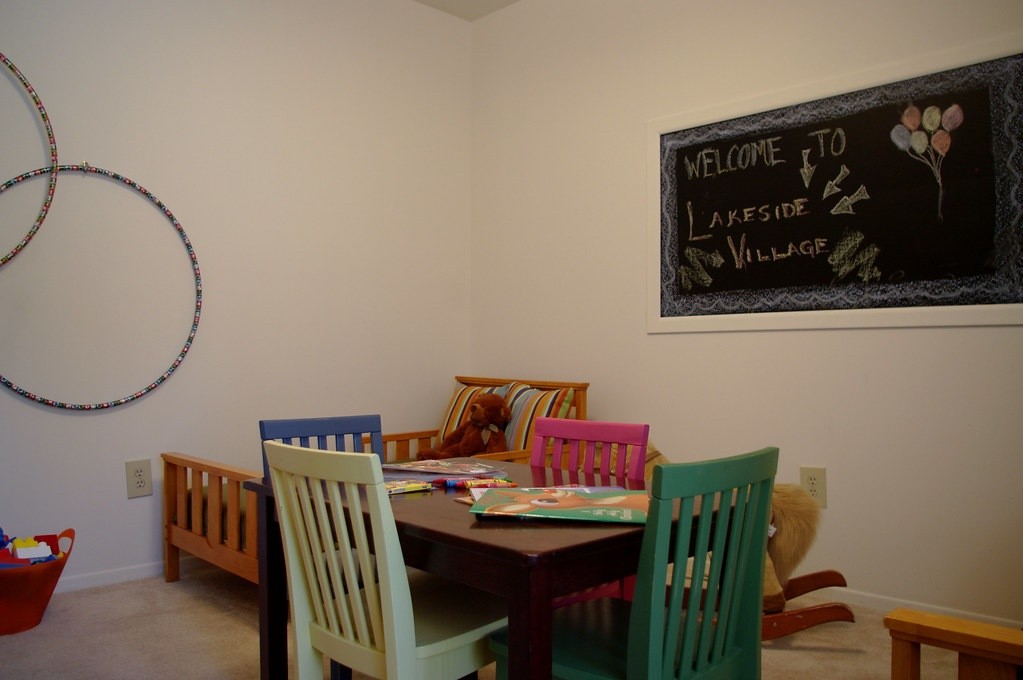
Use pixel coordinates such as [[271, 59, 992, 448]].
[[645, 38, 1023, 336]]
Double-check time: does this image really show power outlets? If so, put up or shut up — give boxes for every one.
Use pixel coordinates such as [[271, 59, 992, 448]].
[[798, 465, 829, 509], [125, 458, 153, 500]]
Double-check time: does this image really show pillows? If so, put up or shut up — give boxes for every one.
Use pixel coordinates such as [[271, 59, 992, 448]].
[[503, 381, 575, 452], [434, 381, 510, 451]]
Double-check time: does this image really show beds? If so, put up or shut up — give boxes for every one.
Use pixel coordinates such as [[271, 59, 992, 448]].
[[161, 376, 590, 585]]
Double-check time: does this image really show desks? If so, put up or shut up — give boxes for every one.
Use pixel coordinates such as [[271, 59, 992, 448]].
[[241, 457, 754, 679]]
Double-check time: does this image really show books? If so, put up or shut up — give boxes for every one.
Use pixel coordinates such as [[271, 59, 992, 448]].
[[381, 458, 508, 481], [454, 484, 649, 524]]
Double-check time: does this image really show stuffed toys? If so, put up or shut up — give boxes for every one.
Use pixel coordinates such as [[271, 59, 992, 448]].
[[581, 437, 818, 612], [416, 394, 512, 461]]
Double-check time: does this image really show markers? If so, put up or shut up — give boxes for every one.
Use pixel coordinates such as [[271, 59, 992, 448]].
[[433, 475, 518, 489]]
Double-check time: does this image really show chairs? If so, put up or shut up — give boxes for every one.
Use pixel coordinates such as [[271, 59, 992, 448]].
[[529, 417, 650, 608], [261, 440, 509, 680], [489, 446, 779, 680], [259, 414, 386, 680]]
